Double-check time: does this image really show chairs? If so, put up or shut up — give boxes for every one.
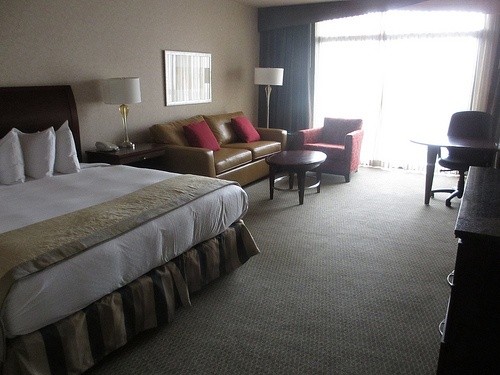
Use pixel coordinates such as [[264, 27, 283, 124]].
[[430, 110, 499, 207]]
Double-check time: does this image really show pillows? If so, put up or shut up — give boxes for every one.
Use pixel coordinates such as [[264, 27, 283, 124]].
[[181, 120, 221, 152], [230, 116, 261, 143], [37, 119, 80, 173], [0, 128, 26, 185], [13, 126, 56, 179]]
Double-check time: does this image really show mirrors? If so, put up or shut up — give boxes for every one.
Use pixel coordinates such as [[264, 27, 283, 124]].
[[164, 50, 211, 107]]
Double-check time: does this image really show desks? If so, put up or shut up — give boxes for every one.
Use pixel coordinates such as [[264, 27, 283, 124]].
[[409, 136, 500, 205]]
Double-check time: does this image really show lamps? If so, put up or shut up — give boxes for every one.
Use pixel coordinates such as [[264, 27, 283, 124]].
[[100, 76, 142, 148], [254, 67, 285, 129]]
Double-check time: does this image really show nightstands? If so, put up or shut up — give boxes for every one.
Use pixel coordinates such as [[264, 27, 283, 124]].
[[84, 142, 174, 172]]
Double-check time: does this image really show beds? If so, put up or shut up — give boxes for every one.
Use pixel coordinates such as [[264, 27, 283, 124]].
[[0, 83, 262, 375]]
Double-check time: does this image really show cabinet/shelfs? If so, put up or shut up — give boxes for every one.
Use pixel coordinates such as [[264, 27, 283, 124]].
[[436, 165, 500, 375]]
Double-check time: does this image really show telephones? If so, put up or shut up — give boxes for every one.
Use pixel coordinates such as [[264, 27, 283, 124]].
[[95, 141, 119, 151]]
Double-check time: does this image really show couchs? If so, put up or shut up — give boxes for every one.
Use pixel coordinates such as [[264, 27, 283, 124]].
[[148, 110, 288, 187], [297, 117, 364, 183]]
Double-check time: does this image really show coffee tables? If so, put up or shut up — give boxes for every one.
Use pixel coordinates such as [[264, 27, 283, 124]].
[[265, 150, 328, 205]]
